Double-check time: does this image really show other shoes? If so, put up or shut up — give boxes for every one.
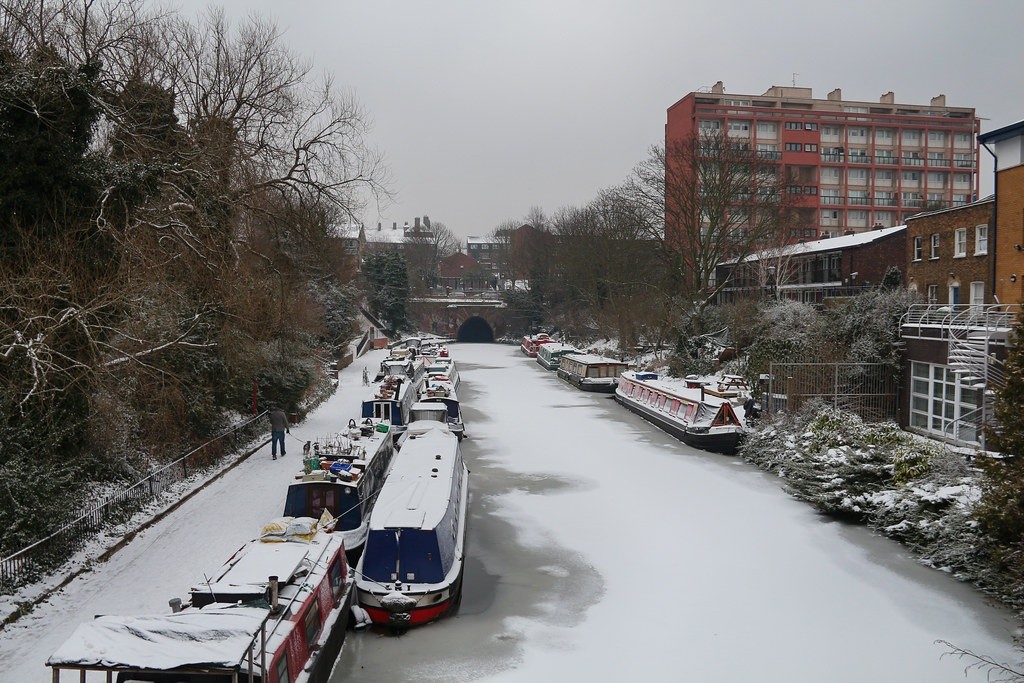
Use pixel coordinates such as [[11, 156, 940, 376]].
[[281, 451, 286, 456], [273, 455, 277, 460]]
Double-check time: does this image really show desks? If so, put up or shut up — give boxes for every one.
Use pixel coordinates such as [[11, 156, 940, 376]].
[[721, 375, 744, 389]]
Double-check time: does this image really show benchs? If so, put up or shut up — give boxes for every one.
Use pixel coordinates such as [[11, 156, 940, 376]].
[[737, 381, 748, 390], [717, 381, 730, 391]]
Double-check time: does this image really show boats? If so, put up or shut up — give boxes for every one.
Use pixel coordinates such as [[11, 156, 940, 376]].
[[520, 333, 556, 358], [556, 353, 629, 392], [46, 527, 356, 683], [360, 338, 467, 442], [354, 419, 470, 635], [284, 416, 396, 570], [615, 370, 745, 445], [537, 343, 586, 371]]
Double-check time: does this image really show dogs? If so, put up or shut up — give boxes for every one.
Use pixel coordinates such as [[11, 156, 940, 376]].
[[312, 442, 319, 451], [303, 441, 311, 454]]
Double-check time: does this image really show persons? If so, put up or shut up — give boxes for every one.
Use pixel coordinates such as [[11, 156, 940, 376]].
[[270, 405, 289, 460]]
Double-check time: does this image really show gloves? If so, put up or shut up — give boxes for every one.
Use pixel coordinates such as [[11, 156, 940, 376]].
[[286, 429, 289, 434]]
